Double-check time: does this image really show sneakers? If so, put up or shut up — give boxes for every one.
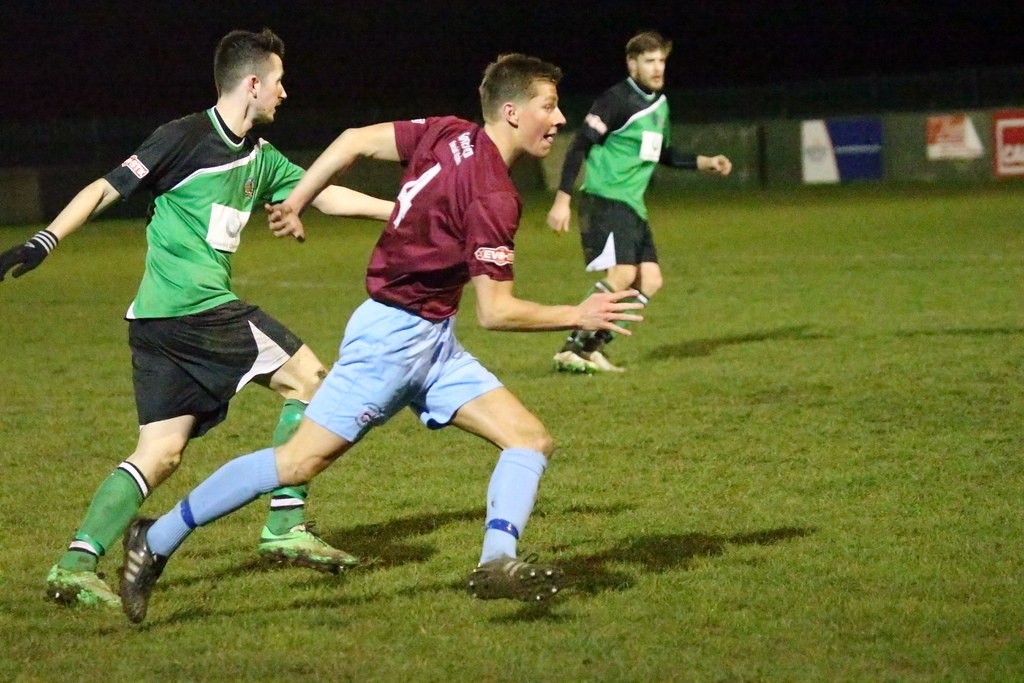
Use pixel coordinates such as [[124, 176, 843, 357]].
[[552, 350, 599, 374], [579, 349, 624, 373], [255, 520, 359, 574], [464, 552, 568, 603], [116, 516, 168, 624], [45, 562, 122, 610]]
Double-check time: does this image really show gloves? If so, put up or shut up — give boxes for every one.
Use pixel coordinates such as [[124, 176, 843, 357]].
[[0, 230, 60, 282]]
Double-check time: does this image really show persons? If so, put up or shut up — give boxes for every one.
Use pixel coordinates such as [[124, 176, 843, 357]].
[[1, 31, 395, 610], [115, 55, 644, 623], [553, 32, 733, 372]]
